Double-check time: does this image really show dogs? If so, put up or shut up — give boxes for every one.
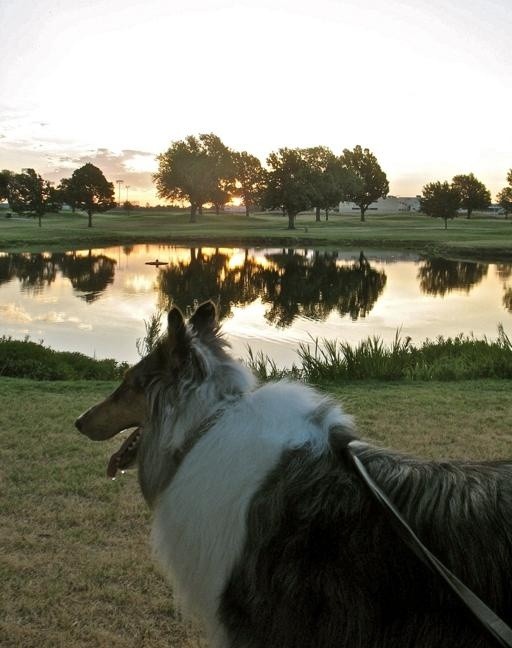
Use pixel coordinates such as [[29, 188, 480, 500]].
[[73, 299, 511, 643]]
